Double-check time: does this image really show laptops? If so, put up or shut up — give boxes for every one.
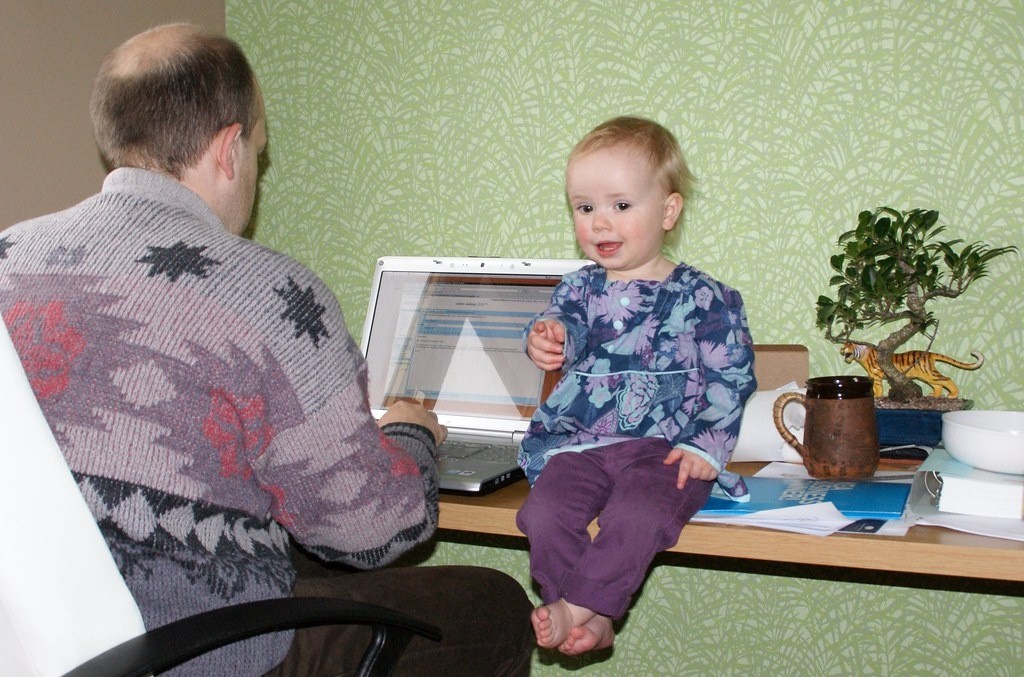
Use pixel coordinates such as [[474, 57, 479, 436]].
[[360, 256, 597, 494]]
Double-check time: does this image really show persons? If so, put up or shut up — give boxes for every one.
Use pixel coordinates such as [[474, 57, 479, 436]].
[[515, 117, 759, 656], [0, 22, 539, 677]]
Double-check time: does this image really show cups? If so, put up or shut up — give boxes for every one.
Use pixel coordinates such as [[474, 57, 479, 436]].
[[773, 375, 881, 482]]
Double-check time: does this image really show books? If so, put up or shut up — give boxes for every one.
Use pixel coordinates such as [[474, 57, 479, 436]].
[[903, 442, 1024, 542]]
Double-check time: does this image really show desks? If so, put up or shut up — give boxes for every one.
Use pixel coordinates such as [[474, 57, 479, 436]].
[[434, 478, 1024, 596]]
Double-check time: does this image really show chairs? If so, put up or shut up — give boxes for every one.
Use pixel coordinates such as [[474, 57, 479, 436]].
[[0, 314, 443, 677]]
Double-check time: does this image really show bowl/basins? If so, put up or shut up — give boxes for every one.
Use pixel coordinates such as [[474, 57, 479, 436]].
[[941, 410, 1024, 475]]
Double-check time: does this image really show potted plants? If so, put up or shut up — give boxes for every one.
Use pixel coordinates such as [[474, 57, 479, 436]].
[[814, 206, 1019, 461]]
